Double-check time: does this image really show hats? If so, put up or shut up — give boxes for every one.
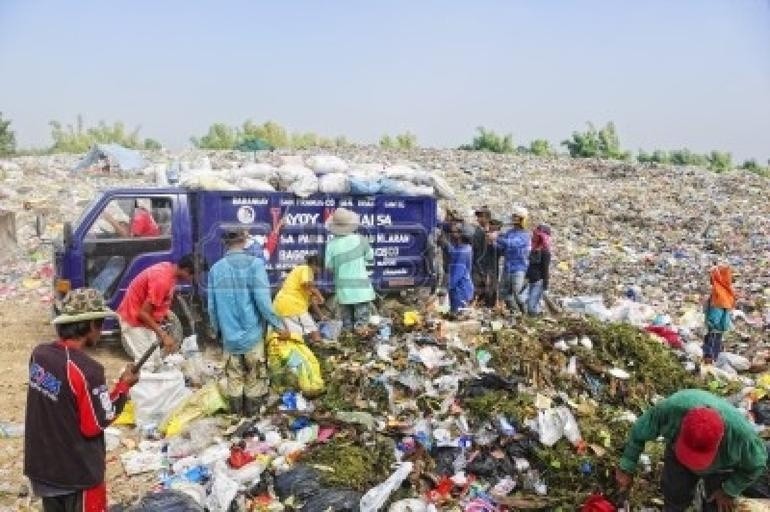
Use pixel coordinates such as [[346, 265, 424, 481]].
[[673, 407, 724, 471], [325, 208, 359, 235], [54, 288, 112, 324]]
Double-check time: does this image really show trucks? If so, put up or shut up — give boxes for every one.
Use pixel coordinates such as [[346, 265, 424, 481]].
[[33, 184, 450, 360]]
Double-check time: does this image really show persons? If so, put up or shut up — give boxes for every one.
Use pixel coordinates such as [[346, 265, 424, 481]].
[[207, 229, 291, 418], [263, 254, 326, 345], [22, 286, 141, 512], [115, 252, 196, 376], [702, 263, 734, 362], [613, 387, 768, 511], [324, 207, 376, 333], [436, 204, 552, 317], [101, 198, 161, 239]]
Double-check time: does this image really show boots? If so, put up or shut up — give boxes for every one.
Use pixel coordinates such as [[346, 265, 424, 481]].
[[229, 395, 260, 415]]
